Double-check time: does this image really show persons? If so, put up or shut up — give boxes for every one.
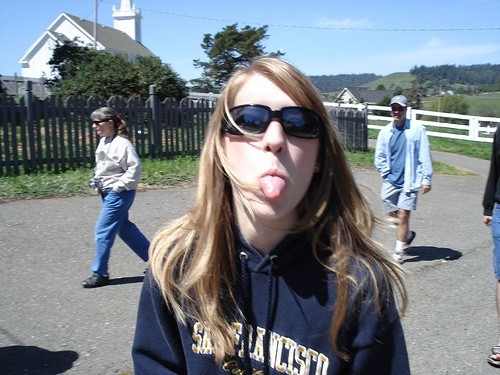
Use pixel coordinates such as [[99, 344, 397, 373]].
[[374, 95, 434, 263], [130, 58, 411, 375], [81, 107, 152, 287], [482, 122, 500, 368]]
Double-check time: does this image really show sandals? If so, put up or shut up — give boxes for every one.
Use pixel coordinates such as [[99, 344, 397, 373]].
[[488, 345, 500, 366]]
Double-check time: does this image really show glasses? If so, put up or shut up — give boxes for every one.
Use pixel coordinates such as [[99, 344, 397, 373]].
[[219, 104, 325, 138], [392, 107, 405, 111], [92, 119, 113, 126]]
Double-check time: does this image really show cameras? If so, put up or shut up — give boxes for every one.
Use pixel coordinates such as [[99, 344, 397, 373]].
[[89, 178, 100, 189]]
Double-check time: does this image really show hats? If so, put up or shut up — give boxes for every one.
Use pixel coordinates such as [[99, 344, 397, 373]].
[[389, 95, 408, 107]]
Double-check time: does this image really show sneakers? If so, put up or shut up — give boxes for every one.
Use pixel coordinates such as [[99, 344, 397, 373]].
[[392, 249, 409, 266], [81, 273, 109, 288], [407, 230, 417, 246]]
